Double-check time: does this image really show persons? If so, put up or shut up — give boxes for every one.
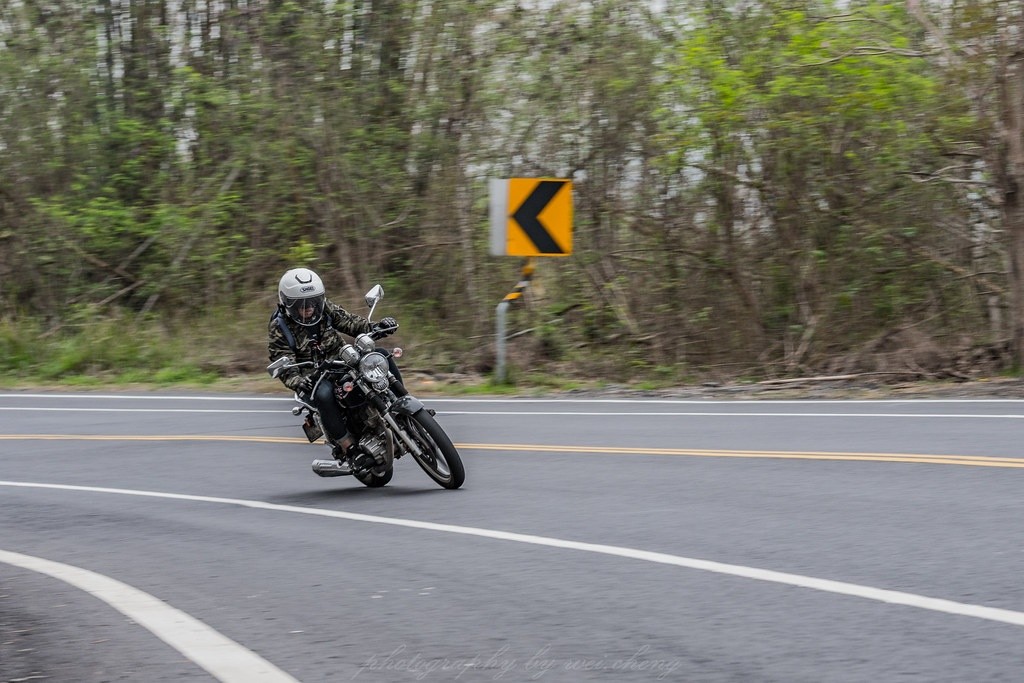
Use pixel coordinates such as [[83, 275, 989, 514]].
[[268, 267, 436, 476]]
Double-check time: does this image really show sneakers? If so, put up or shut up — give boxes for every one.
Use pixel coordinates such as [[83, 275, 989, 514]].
[[424, 409, 437, 417], [344, 445, 365, 466]]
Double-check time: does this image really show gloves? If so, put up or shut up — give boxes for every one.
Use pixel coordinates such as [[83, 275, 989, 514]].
[[298, 373, 312, 390], [377, 315, 398, 335]]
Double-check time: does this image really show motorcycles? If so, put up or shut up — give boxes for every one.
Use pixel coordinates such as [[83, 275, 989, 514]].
[[265, 282, 467, 489]]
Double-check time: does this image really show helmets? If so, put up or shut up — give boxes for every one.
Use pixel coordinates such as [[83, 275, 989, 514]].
[[278, 267, 326, 326]]
